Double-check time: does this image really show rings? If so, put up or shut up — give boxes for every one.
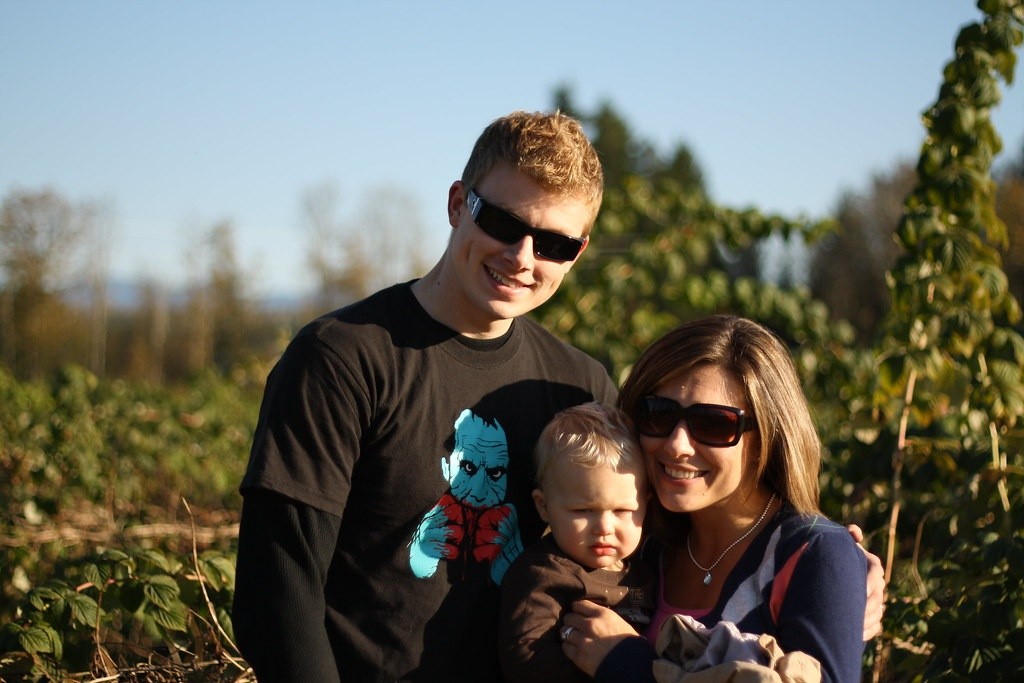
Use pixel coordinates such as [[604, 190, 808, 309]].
[[561, 627, 573, 640]]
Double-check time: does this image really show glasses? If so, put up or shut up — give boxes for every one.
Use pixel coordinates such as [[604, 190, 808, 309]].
[[465, 188, 585, 261], [631, 395, 757, 447]]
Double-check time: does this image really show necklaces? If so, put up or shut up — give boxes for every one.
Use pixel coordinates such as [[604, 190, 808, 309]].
[[687, 491, 776, 585]]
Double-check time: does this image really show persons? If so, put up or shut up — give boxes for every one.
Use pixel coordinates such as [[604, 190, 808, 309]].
[[232, 108, 886, 683]]
